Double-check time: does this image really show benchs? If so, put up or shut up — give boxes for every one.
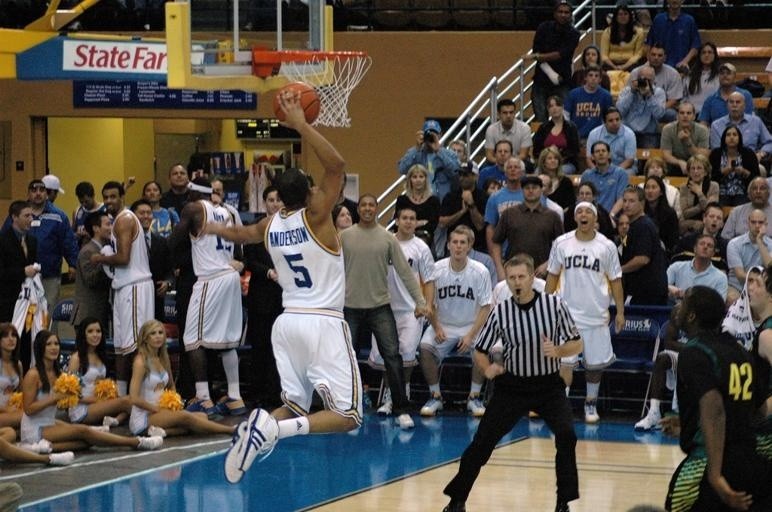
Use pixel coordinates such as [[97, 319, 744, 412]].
[[530, 45, 772, 189]]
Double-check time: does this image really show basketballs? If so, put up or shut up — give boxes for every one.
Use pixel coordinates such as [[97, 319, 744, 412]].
[[274, 82, 319, 124]]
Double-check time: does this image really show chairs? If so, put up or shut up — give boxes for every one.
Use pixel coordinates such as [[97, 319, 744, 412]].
[[46, 290, 252, 389]]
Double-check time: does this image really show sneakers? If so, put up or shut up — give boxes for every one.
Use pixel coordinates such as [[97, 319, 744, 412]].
[[16, 396, 278, 484]]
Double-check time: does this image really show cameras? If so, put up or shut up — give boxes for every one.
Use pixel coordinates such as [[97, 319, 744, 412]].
[[638, 78, 647, 87], [423, 130, 434, 142]]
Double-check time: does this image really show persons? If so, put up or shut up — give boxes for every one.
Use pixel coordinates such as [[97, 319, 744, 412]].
[[657, 285, 772, 512], [482, 95, 582, 179], [0, 164, 283, 466], [747, 260, 772, 472], [211, 90, 363, 482], [566, 0, 771, 303], [441, 252, 583, 510], [546, 201, 624, 424], [522, 4, 581, 121], [370, 205, 438, 415], [335, 121, 582, 282], [633, 299, 720, 431], [419, 226, 492, 418], [340, 193, 427, 429]]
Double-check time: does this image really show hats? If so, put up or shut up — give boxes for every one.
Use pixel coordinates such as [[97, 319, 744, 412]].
[[423, 120, 442, 134], [41, 174, 65, 194], [719, 63, 737, 72], [30, 180, 45, 189]]
